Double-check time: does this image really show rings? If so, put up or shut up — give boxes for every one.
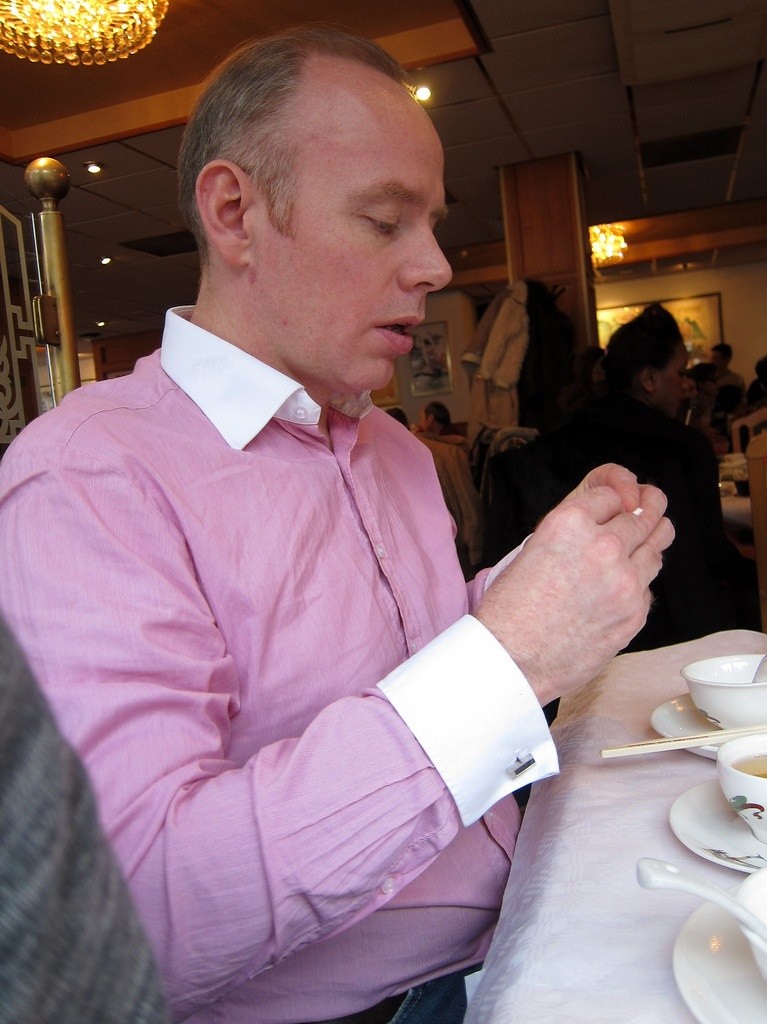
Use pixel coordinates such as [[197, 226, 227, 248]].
[[632, 506, 644, 516]]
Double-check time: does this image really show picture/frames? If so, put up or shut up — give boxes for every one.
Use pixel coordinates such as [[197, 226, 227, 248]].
[[370, 356, 403, 410], [407, 321, 455, 399], [597, 292, 724, 369]]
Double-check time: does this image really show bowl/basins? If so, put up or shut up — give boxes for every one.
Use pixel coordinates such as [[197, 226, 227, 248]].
[[732, 866, 766, 953], [716, 735, 767, 843], [679, 654, 767, 730]]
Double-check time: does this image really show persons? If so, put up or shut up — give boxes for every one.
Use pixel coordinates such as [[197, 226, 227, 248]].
[[418, 401, 464, 436], [0, 608, 175, 1024], [0, 21, 675, 1024], [530, 299, 762, 632], [682, 343, 744, 455], [747, 355, 767, 411]]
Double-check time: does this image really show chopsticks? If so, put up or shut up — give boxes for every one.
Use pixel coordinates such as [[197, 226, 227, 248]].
[[600, 731, 747, 759]]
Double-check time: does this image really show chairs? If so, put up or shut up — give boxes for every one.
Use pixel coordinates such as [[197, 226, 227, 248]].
[[731, 406, 767, 456], [747, 431, 767, 637]]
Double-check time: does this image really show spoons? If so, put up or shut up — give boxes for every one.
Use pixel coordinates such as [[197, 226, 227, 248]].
[[635, 857, 767, 942]]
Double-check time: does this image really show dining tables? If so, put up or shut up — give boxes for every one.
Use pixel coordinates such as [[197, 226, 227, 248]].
[[464, 628, 767, 1024], [719, 476, 753, 534]]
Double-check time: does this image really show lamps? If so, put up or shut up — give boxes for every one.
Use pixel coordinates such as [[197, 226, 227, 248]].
[[0, 0, 170, 66]]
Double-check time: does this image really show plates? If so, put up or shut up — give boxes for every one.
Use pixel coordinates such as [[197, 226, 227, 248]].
[[668, 780, 767, 874], [650, 693, 718, 760], [672, 885, 765, 1023]]
